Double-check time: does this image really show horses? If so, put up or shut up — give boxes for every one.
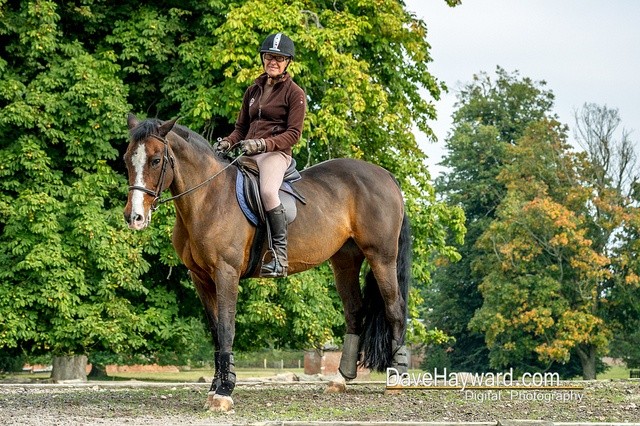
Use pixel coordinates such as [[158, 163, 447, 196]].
[[120, 109, 415, 416]]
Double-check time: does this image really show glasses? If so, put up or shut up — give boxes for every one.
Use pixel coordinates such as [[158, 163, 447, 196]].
[[264, 54, 291, 62]]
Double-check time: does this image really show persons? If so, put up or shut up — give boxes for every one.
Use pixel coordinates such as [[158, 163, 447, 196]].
[[214, 32, 307, 278]]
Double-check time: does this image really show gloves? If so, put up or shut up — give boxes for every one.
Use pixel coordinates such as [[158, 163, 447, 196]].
[[241, 138, 266, 155], [214, 140, 228, 153]]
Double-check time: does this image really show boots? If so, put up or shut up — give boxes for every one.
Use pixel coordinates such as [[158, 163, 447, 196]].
[[261, 203, 288, 276]]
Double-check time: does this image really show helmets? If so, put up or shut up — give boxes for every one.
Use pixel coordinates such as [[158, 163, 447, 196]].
[[261, 33, 295, 57]]
